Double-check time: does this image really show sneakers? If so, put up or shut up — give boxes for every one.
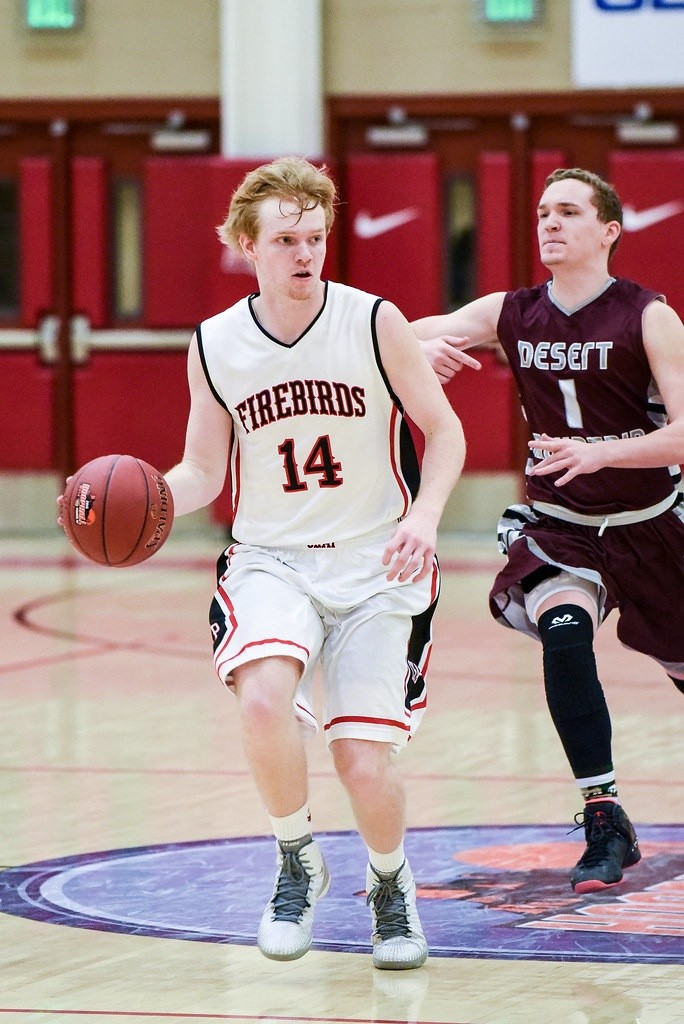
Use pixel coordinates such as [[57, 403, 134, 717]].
[[365, 858, 429, 970], [566, 798, 642, 893], [256, 834, 332, 960]]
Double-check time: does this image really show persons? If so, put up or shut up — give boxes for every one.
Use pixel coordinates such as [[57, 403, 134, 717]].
[[411, 169, 684, 892], [55, 156, 469, 970]]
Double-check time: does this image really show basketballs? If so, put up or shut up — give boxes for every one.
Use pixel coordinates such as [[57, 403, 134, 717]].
[[60, 454, 175, 568]]
